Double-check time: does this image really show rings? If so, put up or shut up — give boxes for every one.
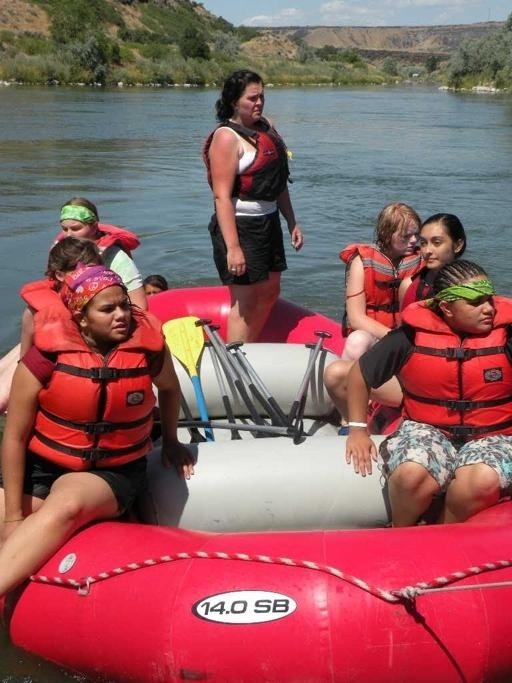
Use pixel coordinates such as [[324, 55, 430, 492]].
[[232, 268, 236, 272]]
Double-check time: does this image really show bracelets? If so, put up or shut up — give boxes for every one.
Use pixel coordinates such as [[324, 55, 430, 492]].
[[346, 421, 369, 429], [2, 518, 25, 523]]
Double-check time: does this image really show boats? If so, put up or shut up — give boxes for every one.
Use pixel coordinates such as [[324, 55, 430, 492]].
[[1, 287, 510, 678]]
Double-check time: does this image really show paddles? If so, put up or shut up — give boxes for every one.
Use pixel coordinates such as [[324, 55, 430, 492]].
[[161, 316, 214, 442]]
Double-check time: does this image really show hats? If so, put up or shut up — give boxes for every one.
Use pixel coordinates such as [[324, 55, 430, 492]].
[[56, 260, 128, 319]]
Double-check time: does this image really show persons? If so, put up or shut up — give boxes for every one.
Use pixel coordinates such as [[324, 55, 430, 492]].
[[342, 195, 423, 364], [344, 260, 512, 528], [200, 69, 304, 345], [20, 236, 103, 361], [0, 262, 194, 595], [143, 274, 167, 295], [0, 197, 148, 418], [317, 214, 469, 436]]
